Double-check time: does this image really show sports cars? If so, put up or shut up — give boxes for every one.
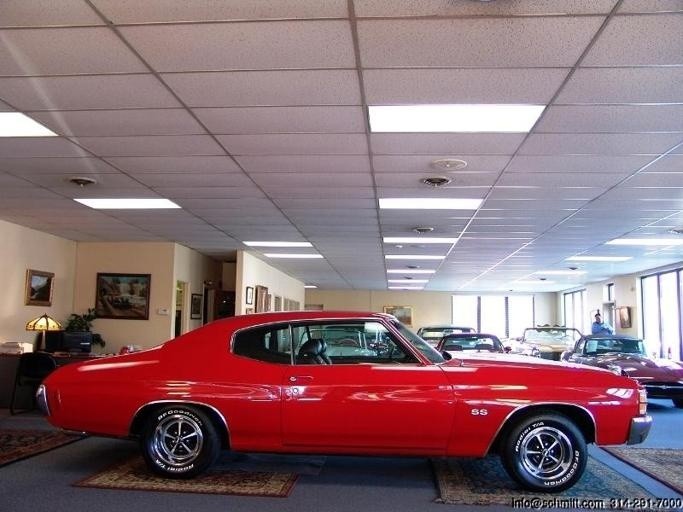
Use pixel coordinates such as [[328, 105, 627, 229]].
[[502, 323, 584, 361]]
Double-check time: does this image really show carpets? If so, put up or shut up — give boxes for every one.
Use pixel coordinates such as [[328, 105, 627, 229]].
[[426, 450, 669, 512], [600, 439, 682, 496], [0, 422, 88, 470], [69, 444, 304, 498]]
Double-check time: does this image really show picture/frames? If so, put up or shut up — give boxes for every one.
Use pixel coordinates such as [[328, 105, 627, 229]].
[[23, 266, 54, 307], [244, 284, 273, 316], [190, 291, 204, 321], [92, 270, 151, 321]]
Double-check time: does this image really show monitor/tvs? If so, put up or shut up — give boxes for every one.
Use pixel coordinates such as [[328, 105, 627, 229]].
[[64, 332, 91, 354]]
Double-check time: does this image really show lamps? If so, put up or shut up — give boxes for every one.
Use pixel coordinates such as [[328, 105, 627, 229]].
[[25, 314, 62, 352]]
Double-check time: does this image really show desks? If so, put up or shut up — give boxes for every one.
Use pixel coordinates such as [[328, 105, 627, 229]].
[[0, 350, 105, 410]]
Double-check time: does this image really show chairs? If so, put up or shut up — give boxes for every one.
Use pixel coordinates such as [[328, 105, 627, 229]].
[[296, 339, 327, 365], [446, 342, 462, 350], [472, 342, 494, 349], [315, 335, 333, 365], [6, 350, 60, 416]]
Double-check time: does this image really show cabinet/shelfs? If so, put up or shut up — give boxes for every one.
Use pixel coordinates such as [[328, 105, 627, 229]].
[[36, 329, 91, 354]]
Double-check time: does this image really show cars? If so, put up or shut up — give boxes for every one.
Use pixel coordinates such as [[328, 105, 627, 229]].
[[560, 334, 683, 409], [285, 329, 377, 357], [415, 325, 476, 351], [433, 332, 512, 356], [313, 325, 388, 354], [35, 311, 652, 495]]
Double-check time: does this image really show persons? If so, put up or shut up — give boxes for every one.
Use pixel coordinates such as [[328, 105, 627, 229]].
[[591, 314, 613, 335]]
